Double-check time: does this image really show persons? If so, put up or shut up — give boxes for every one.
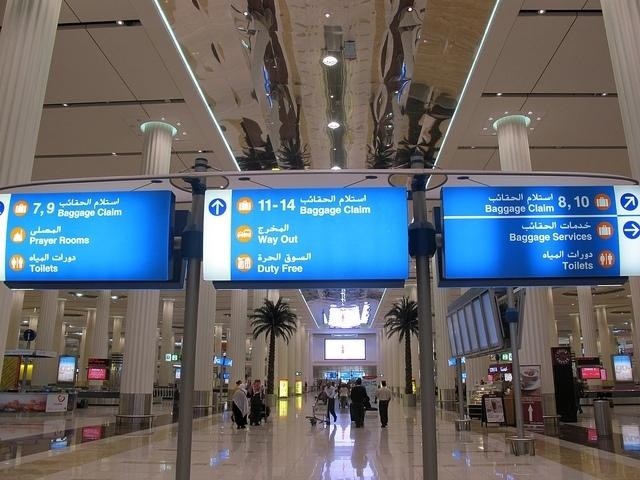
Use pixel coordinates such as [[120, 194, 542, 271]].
[[574, 376, 584, 414], [231, 379, 265, 428], [316, 377, 392, 428]]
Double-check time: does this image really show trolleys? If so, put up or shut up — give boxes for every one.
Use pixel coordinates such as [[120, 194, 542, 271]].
[[305, 396, 331, 426], [250, 396, 271, 426], [338, 397, 349, 409], [350, 402, 366, 425]]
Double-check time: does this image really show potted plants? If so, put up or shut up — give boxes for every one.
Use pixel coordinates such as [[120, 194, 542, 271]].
[[382, 295, 418, 408], [245, 294, 298, 406]]
